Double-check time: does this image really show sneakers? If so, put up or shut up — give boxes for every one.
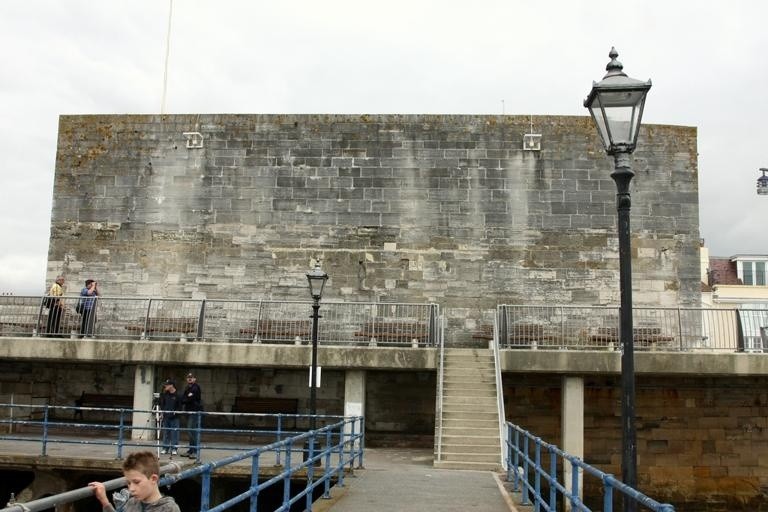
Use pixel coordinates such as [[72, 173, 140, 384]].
[[181, 452, 196, 458], [161, 449, 178, 455]]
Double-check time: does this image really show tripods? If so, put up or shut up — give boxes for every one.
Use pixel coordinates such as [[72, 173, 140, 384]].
[[140, 399, 178, 459]]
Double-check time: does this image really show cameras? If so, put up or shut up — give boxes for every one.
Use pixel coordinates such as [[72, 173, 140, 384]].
[[152, 392, 162, 398]]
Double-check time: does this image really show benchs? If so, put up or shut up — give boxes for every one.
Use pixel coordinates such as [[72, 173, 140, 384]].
[[71, 390, 133, 420], [230, 395, 301, 432]]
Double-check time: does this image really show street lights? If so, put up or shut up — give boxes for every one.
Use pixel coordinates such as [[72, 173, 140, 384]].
[[583, 45, 652, 511], [303, 257, 329, 466], [756, 167, 768, 195]]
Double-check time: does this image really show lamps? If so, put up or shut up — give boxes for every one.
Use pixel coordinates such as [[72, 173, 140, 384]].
[[181, 131, 203, 149], [522, 132, 543, 151]]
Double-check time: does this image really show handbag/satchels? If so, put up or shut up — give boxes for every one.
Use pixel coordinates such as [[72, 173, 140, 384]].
[[76, 303, 85, 313], [43, 298, 57, 308]]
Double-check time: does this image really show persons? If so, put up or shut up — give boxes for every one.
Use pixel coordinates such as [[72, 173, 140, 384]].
[[89, 452, 181, 512], [160, 379, 182, 455], [77, 280, 102, 339], [44, 276, 65, 338], [180, 372, 201, 459]]
